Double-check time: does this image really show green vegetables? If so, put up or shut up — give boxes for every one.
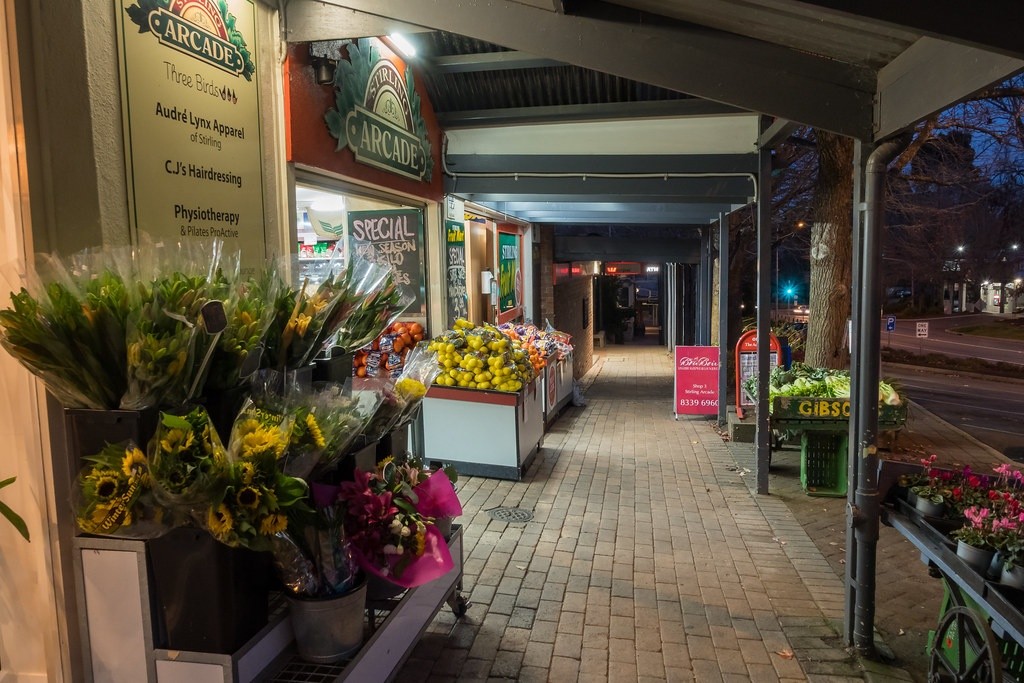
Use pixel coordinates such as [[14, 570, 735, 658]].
[[743, 360, 900, 406]]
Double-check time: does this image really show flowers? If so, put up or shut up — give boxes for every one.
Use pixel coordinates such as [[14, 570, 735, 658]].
[[902, 454, 1024, 566], [0, 236, 463, 588]]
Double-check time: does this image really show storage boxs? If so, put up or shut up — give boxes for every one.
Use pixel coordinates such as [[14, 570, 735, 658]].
[[799, 430, 849, 498], [921, 575, 1024, 681]]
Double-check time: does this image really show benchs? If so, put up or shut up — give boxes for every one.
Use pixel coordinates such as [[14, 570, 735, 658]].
[[593, 330, 606, 348]]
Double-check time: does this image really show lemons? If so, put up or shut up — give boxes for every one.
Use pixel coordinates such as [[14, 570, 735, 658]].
[[428, 319, 538, 392]]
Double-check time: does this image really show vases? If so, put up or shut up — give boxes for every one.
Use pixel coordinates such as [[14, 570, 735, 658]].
[[63, 353, 454, 663], [906, 488, 1024, 589]]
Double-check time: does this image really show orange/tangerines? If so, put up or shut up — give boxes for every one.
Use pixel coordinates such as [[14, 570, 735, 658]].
[[352, 323, 422, 378], [503, 330, 545, 371]]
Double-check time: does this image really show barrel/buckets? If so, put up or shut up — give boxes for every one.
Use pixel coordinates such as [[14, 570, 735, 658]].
[[284, 569, 370, 663]]
